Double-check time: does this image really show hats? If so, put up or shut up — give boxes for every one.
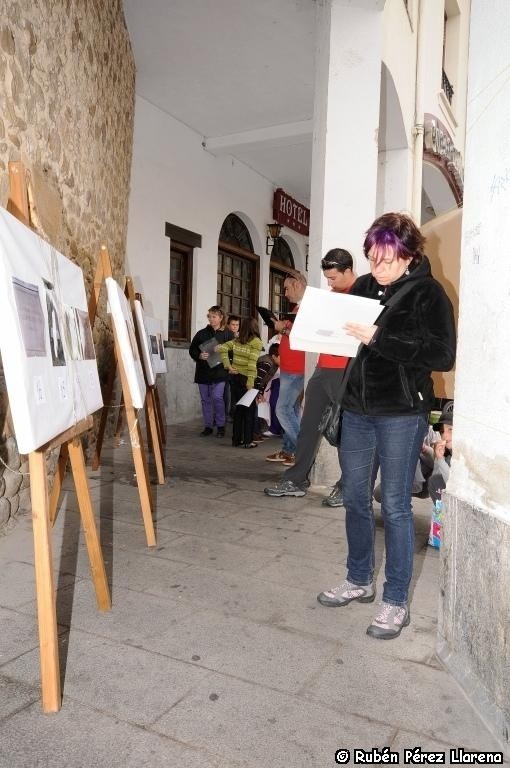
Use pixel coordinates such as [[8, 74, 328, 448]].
[[432, 410, 453, 433]]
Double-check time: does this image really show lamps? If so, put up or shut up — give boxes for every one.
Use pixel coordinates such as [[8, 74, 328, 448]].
[[263, 219, 283, 253]]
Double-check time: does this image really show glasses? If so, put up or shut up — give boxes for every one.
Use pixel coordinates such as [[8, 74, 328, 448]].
[[321, 260, 350, 269]]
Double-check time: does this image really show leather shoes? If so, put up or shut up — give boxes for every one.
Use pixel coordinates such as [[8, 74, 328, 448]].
[[216, 426, 226, 438], [199, 426, 213, 437]]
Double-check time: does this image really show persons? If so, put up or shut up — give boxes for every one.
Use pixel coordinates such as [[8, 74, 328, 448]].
[[428, 414, 453, 505], [266, 271, 307, 466], [189, 306, 285, 448], [318, 213, 456, 640], [374, 424, 442, 503], [264, 249, 357, 507]]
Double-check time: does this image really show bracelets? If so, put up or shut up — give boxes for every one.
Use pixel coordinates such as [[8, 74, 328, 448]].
[[281, 327, 287, 335]]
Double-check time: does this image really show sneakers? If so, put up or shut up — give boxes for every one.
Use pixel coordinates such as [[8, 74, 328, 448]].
[[231, 430, 281, 450], [320, 487, 343, 507], [264, 476, 305, 497], [317, 580, 375, 608], [365, 602, 410, 640], [281, 456, 297, 467], [265, 448, 296, 462]]
